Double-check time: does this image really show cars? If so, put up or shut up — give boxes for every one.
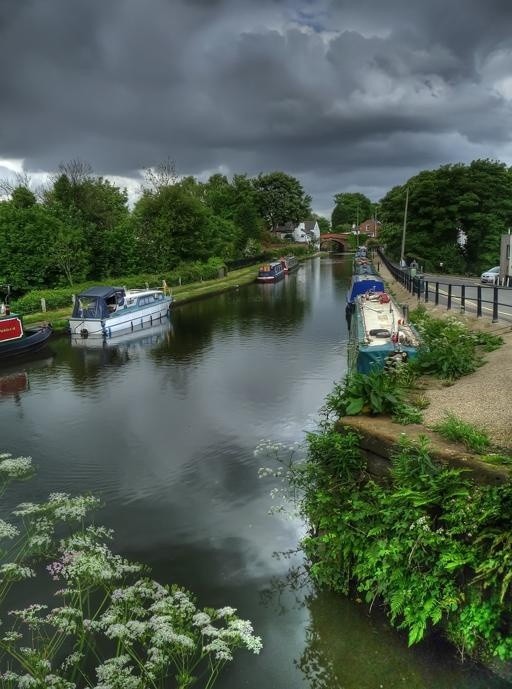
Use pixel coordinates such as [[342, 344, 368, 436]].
[[480, 266, 501, 284]]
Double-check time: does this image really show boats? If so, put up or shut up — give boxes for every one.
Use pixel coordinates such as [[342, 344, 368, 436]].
[[0, 304, 53, 356], [256, 261, 285, 280], [344, 246, 385, 309], [278, 253, 299, 272], [67, 285, 174, 335], [348, 290, 428, 382]]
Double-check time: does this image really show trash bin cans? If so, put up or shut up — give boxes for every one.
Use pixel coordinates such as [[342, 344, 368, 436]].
[[414, 275, 424, 294]]
